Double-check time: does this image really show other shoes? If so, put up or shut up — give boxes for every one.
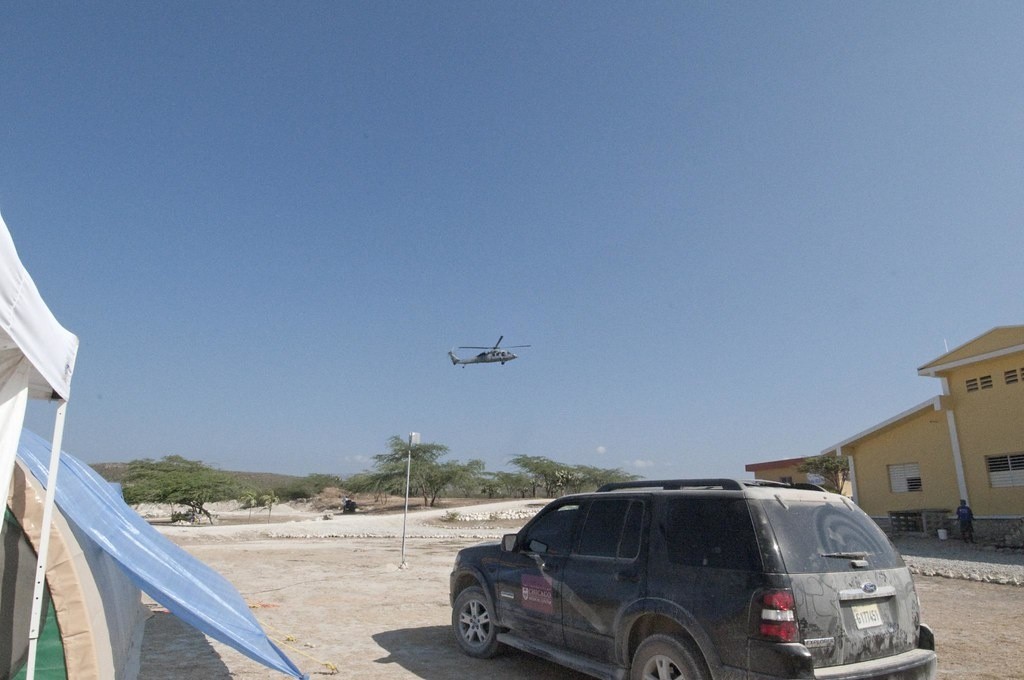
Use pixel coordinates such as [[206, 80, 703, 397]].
[[971, 539, 977, 544]]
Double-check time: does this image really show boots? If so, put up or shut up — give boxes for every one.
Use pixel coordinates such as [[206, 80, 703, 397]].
[[964, 538, 968, 543]]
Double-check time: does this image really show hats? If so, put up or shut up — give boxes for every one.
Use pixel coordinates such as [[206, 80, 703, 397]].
[[960, 499, 966, 505]]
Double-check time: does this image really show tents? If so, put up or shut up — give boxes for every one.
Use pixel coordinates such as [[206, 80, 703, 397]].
[[0, 212, 312, 680]]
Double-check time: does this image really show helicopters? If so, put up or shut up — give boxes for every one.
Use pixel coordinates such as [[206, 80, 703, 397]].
[[447, 335, 531, 368]]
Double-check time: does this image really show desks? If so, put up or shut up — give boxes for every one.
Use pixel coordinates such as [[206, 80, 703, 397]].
[[887, 508, 952, 538]]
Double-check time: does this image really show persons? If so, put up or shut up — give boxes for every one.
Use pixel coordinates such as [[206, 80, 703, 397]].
[[956, 499, 976, 543]]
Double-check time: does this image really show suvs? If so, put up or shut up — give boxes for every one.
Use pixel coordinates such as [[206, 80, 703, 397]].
[[450, 478, 938, 680]]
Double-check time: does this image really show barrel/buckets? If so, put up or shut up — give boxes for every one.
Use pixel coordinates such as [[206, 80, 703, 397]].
[[937, 529, 948, 540]]
